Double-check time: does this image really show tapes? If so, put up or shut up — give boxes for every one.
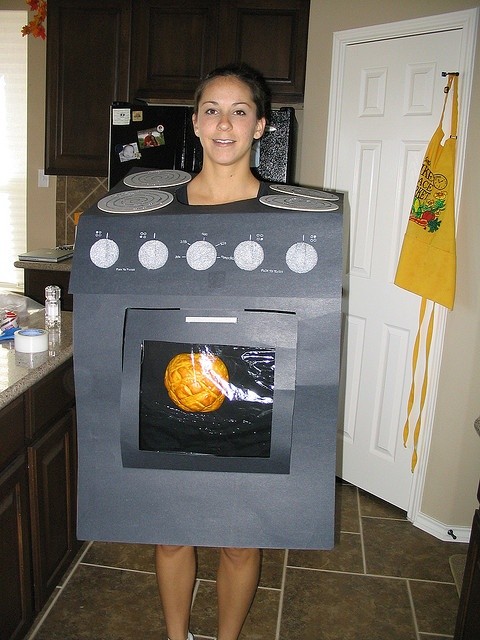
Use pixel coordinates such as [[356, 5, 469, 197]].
[[14, 329, 49, 353]]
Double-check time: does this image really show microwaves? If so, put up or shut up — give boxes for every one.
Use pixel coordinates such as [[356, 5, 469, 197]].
[[108, 103, 295, 192]]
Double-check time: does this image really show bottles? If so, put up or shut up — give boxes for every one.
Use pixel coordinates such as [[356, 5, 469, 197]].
[[44, 285, 61, 330]]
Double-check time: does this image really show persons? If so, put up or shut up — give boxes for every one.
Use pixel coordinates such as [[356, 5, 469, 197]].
[[143, 131, 152, 147], [147, 136, 160, 147], [138, 61, 269, 640], [120, 145, 136, 162]]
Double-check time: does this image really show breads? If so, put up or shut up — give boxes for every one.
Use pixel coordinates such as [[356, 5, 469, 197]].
[[164, 353, 230, 413]]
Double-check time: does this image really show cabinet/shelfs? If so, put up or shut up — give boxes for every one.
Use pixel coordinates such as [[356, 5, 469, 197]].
[[24, 356, 85, 620], [44, 2, 130, 177], [0, 388, 34, 639], [130, 2, 311, 109], [24, 270, 74, 312]]
